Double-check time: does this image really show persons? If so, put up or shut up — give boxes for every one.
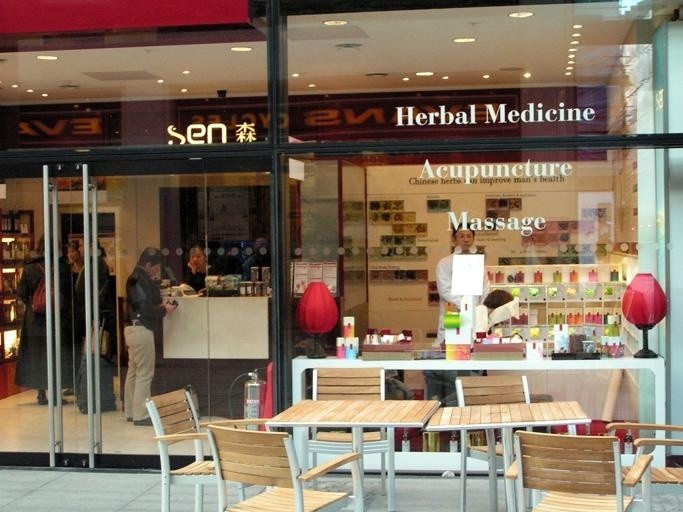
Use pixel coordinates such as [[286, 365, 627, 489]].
[[185, 244, 218, 293], [436, 222, 491, 340], [15, 234, 71, 405], [75, 234, 116, 414], [125, 247, 176, 425], [63, 240, 77, 397]]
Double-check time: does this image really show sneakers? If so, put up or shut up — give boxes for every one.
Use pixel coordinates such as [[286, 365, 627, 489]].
[[126, 417, 133, 422], [133, 417, 153, 426]]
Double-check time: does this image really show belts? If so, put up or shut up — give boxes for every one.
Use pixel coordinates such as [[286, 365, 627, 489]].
[[127, 320, 143, 326]]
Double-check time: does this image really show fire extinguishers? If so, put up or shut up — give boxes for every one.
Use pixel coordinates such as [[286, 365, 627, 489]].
[[226, 367, 267, 433]]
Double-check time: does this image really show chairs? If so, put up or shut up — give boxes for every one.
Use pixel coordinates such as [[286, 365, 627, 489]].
[[512, 430, 650, 512], [452, 374, 534, 511], [608, 423, 683, 485], [305, 369, 390, 494], [207, 420, 362, 512], [146, 389, 270, 512]]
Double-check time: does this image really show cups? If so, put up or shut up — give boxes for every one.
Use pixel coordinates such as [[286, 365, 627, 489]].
[[343, 317, 354, 338], [346, 336, 356, 360], [336, 338, 346, 359]]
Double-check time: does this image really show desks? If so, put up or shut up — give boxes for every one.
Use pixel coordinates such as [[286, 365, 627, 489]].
[[429, 400, 590, 511], [266, 400, 442, 512]]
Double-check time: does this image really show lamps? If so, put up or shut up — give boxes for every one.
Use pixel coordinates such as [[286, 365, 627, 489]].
[[622, 272, 667, 359], [296, 282, 337, 359]]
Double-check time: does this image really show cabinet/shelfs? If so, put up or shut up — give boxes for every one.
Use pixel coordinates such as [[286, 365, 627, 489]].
[[0, 208, 35, 401]]
